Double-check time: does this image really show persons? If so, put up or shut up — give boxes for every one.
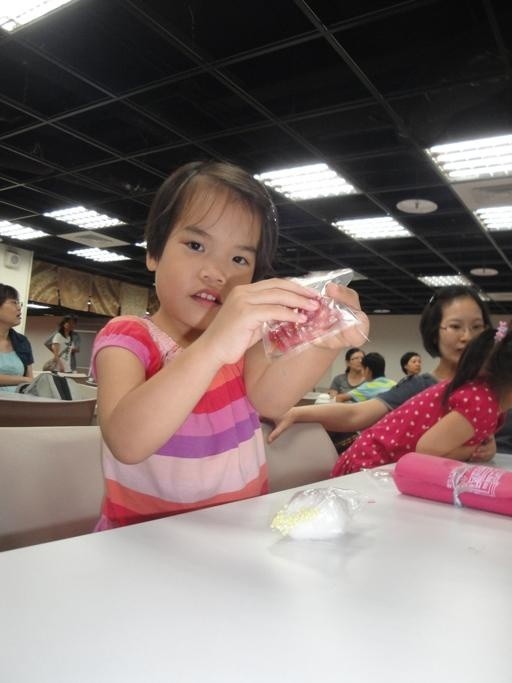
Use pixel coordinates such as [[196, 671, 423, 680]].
[[0, 281, 36, 396], [330, 346, 370, 404], [52, 316, 74, 373], [43, 316, 82, 373], [330, 314, 512, 489], [335, 351, 398, 404], [258, 283, 511, 478], [400, 351, 423, 377], [80, 158, 371, 534]]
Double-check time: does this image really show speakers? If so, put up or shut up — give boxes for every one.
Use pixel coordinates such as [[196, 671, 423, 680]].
[[4, 251, 20, 269]]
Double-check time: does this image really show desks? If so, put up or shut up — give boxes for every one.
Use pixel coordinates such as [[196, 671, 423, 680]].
[[0, 454, 509, 683]]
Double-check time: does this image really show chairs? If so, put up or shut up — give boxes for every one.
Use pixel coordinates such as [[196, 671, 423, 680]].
[[1, 423, 339, 551]]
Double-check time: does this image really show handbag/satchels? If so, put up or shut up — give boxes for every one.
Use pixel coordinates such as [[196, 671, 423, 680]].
[[43, 330, 73, 357], [43, 358, 64, 372]]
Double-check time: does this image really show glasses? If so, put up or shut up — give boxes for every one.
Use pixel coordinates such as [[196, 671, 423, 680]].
[[439, 321, 488, 335], [1, 300, 24, 307]]
